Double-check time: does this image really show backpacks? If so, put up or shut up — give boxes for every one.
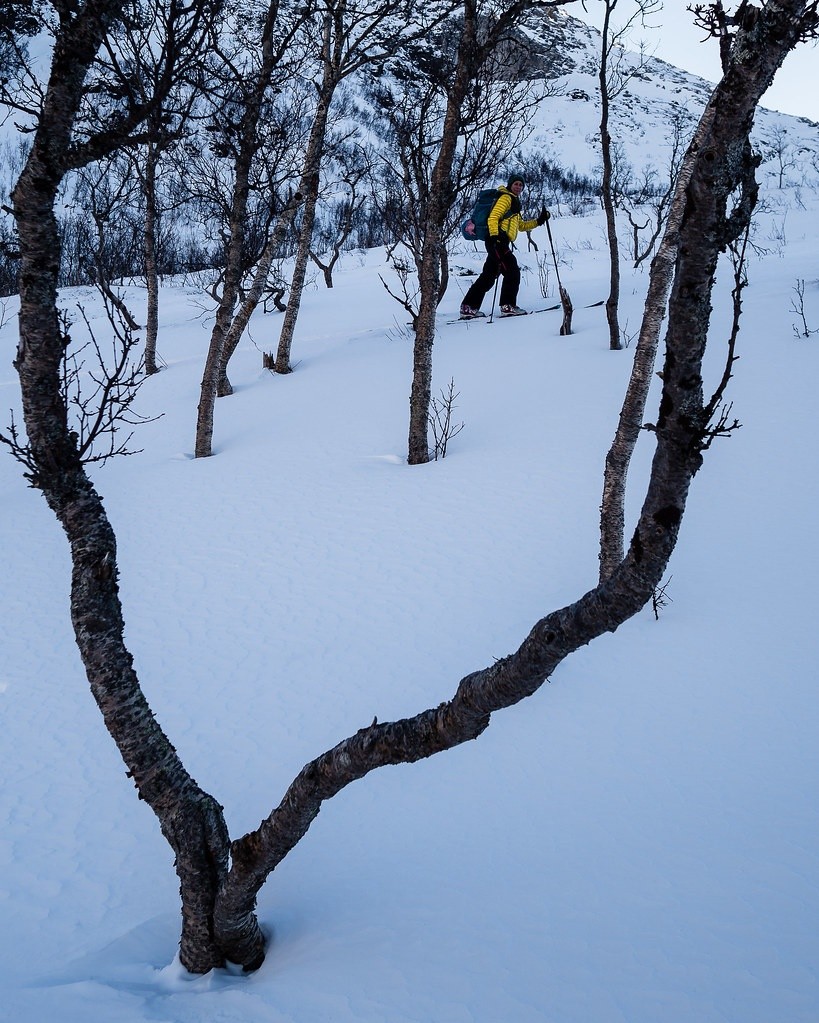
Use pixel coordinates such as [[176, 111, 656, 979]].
[[472, 189, 522, 240]]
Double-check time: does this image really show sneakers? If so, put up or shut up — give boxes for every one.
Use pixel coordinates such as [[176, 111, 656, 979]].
[[459, 304, 485, 319], [501, 303, 526, 316]]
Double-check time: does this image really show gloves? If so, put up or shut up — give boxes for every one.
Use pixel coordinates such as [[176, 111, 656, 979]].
[[538, 208, 547, 226], [490, 235, 503, 249]]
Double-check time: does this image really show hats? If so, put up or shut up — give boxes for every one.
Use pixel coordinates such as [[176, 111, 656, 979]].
[[508, 173, 526, 188]]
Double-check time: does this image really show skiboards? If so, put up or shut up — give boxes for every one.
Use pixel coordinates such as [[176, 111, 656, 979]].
[[406, 300, 605, 325]]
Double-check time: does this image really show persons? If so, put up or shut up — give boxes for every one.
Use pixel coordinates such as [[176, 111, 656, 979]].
[[461, 176, 550, 319]]
